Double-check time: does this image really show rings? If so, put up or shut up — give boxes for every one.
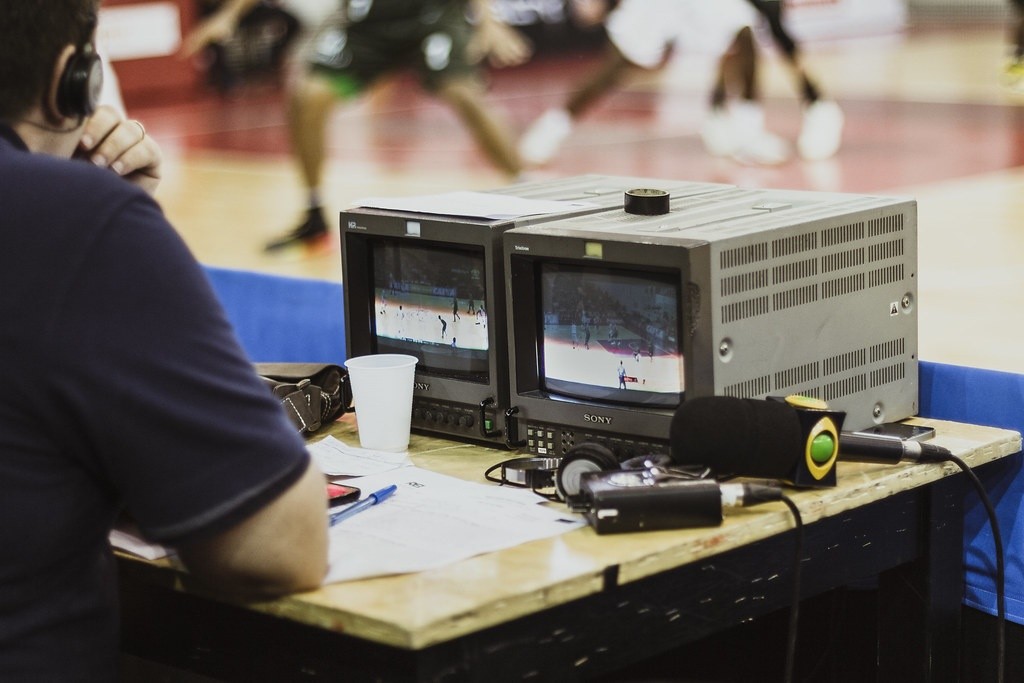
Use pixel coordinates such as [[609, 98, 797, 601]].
[[129, 120, 147, 141]]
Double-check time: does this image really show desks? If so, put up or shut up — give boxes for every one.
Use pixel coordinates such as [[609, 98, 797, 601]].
[[101, 364, 1024, 683]]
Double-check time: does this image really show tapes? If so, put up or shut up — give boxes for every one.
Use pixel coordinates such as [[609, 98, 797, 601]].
[[624, 188, 670, 215]]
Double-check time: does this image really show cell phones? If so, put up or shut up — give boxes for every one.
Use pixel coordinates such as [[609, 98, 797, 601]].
[[851, 422, 936, 443]]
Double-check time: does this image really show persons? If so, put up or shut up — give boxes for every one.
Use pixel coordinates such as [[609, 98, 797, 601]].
[[171, 0, 535, 252], [517, 0, 847, 164], [541, 273, 680, 392], [372, 246, 488, 351], [1003, 0, 1024, 90], [0, 0, 333, 683]]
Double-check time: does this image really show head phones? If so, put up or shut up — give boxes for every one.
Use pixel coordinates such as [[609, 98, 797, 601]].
[[56, 17, 103, 120], [501, 443, 673, 501]]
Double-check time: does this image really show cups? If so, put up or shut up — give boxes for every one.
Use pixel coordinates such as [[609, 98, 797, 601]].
[[343, 355, 420, 451]]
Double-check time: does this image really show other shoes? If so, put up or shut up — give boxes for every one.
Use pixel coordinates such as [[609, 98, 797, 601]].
[[801, 101, 841, 158], [268, 222, 329, 252], [520, 104, 570, 167]]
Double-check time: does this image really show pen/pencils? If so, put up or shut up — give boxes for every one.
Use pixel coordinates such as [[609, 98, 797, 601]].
[[329, 484, 398, 527]]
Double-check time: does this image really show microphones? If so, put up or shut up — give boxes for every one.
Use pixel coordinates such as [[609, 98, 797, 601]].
[[665, 392, 952, 488]]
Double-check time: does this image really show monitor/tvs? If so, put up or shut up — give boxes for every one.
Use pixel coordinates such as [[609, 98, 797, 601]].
[[339, 175, 917, 467]]
